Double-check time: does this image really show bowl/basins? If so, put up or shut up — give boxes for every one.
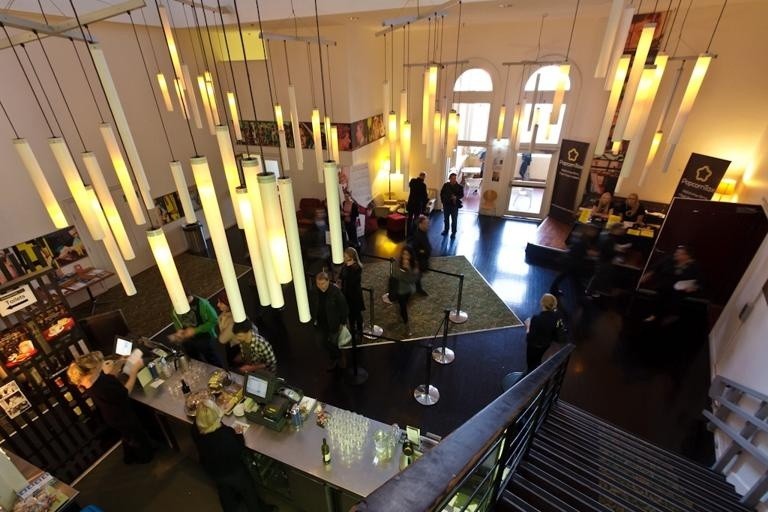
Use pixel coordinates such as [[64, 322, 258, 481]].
[[184, 367, 232, 417]]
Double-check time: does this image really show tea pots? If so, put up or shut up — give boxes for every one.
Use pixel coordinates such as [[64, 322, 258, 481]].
[[400, 439, 414, 471]]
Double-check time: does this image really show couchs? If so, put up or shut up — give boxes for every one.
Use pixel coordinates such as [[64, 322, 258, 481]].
[[579, 192, 668, 224]]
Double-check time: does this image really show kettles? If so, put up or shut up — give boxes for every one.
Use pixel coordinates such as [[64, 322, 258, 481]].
[[173, 351, 188, 373]]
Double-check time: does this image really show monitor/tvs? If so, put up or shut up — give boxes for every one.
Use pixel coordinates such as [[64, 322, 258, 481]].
[[243, 371, 280, 402], [113, 336, 134, 357]]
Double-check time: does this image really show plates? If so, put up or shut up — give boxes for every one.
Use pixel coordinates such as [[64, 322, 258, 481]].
[[47, 327, 65, 337], [63, 318, 70, 326], [13, 354, 29, 363], [232, 403, 245, 417]]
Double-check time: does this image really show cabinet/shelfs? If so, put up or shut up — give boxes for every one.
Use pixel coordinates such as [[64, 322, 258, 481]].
[[635, 197, 767, 336]]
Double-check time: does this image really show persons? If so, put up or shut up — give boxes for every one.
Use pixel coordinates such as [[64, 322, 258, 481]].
[[314, 190, 359, 247], [336, 125, 351, 150], [382, 247, 420, 339], [354, 123, 366, 145], [523, 293, 558, 373], [233, 320, 277, 377], [168, 283, 217, 367], [215, 292, 257, 368], [334, 247, 366, 345], [65, 351, 152, 464], [519, 152, 531, 181], [642, 246, 702, 324], [577, 190, 645, 298], [439, 173, 463, 238], [404, 171, 428, 233], [413, 215, 431, 296], [313, 272, 348, 371], [299, 123, 312, 148], [193, 399, 261, 511], [473, 151, 484, 193]]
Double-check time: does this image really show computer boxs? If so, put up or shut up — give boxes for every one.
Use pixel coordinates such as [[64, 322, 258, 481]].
[[245, 396, 291, 432]]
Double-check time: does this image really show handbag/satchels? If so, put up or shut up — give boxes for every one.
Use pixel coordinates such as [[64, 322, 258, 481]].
[[556, 318, 569, 344]]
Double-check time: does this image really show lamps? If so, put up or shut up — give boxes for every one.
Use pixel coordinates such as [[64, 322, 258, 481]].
[[376, 0, 727, 192], [715, 178, 737, 202], [0, 0, 345, 324]]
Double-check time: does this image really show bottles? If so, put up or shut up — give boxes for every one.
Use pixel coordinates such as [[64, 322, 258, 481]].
[[180, 379, 191, 399], [290, 407, 301, 427], [321, 437, 331, 464], [147, 362, 160, 381]]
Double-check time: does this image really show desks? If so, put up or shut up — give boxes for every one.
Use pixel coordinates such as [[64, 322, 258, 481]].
[[565, 221, 660, 264], [18, 267, 115, 317], [1, 447, 79, 512], [43, 317, 75, 344], [6, 348, 40, 370]]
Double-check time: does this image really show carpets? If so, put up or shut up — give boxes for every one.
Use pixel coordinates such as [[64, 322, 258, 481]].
[[337, 255, 524, 349], [0, 252, 251, 342]]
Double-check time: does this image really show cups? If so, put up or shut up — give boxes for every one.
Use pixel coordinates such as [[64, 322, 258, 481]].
[[168, 361, 207, 396], [373, 423, 402, 464], [327, 407, 368, 453]]
